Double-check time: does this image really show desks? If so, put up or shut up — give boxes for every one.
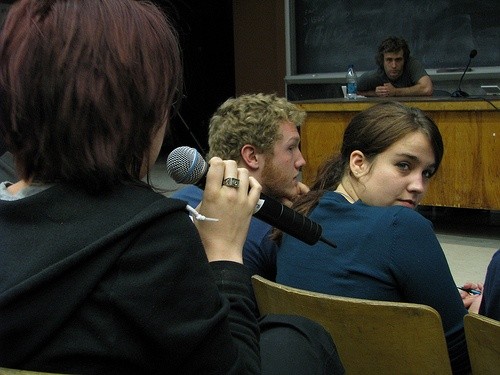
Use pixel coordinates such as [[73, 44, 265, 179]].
[[288, 95, 500, 217]]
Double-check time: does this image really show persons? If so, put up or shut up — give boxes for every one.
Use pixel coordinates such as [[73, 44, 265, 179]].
[[272, 100, 484, 375], [165, 93, 311, 282], [0, 0, 345, 375], [355, 36, 433, 97]]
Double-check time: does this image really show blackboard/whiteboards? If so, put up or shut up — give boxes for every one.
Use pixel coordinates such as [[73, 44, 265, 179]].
[[285, 0, 500, 79]]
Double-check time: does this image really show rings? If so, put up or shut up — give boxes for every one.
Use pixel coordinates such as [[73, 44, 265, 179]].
[[223, 178, 239, 187]]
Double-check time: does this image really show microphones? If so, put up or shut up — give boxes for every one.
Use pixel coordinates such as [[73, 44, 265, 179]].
[[451, 49, 478, 98], [166, 146, 338, 249]]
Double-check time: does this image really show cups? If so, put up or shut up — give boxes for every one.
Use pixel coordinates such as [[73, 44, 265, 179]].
[[342, 85, 347, 98]]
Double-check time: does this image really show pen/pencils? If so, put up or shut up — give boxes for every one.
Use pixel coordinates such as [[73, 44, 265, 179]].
[[457, 286, 481, 296]]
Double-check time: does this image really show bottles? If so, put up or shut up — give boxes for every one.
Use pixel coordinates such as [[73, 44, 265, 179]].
[[346, 64, 357, 100]]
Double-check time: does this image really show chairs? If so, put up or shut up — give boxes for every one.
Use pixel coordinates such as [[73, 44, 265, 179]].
[[463, 311, 500, 375], [251, 274, 453, 375]]
